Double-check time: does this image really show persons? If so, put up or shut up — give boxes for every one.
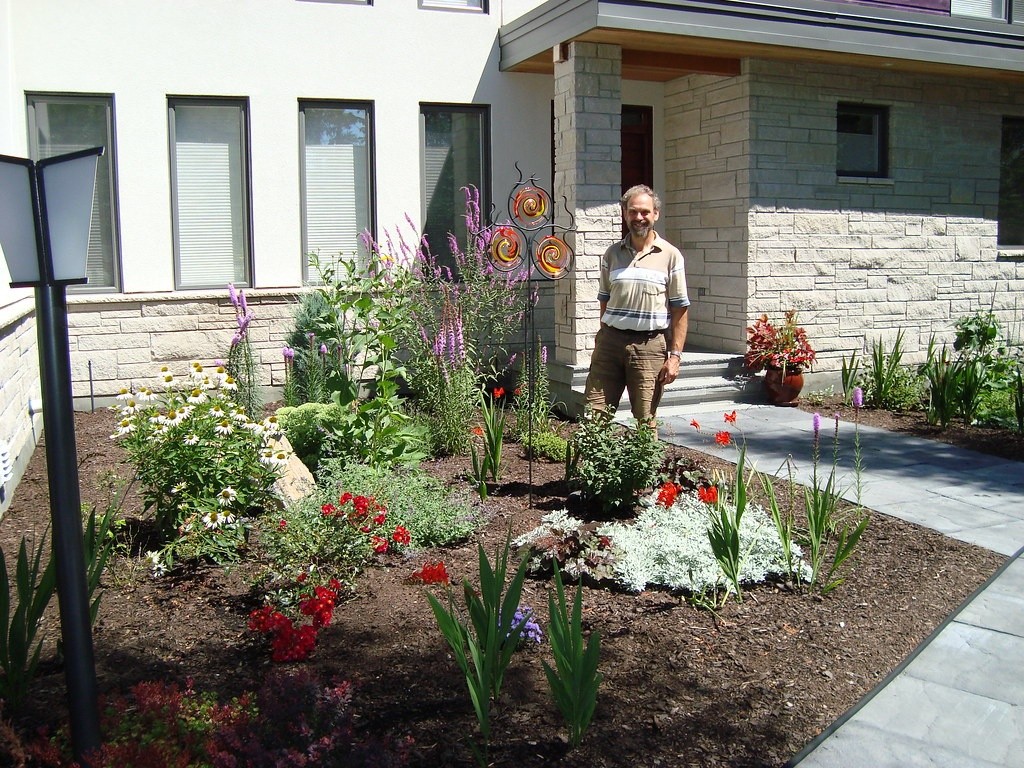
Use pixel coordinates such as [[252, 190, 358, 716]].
[[585, 184, 690, 443]]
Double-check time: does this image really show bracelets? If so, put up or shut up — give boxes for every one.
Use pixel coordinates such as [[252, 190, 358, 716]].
[[671, 350, 682, 361]]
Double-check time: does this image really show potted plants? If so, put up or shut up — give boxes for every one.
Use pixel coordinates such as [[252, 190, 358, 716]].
[[737, 308, 827, 403]]
